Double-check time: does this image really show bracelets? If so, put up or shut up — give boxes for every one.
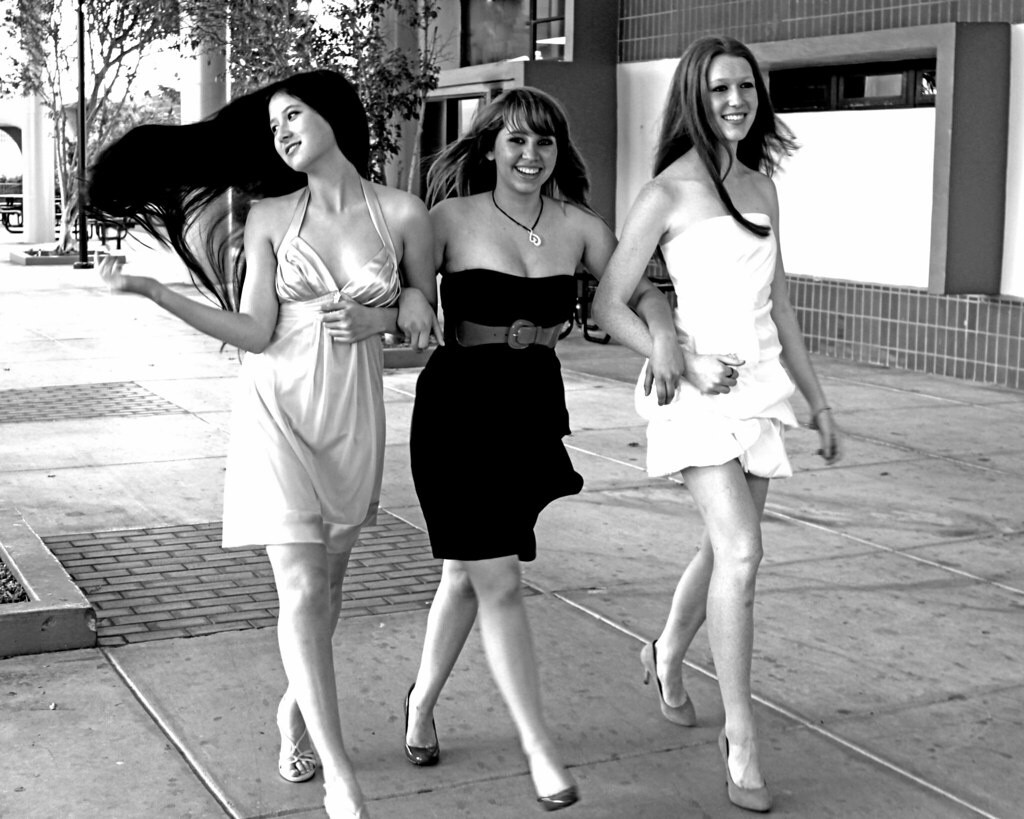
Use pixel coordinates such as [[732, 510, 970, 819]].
[[809, 407, 833, 431]]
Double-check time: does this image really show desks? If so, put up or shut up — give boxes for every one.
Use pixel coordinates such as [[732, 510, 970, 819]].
[[1, 193, 62, 225]]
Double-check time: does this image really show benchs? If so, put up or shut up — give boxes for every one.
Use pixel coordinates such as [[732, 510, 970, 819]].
[[0, 202, 133, 249]]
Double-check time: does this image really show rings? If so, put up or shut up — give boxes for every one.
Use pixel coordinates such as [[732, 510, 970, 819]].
[[726, 367, 734, 378]]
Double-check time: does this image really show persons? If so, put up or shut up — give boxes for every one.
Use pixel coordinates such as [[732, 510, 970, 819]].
[[72, 69, 438, 819], [592, 36, 840, 809], [396, 87, 683, 810]]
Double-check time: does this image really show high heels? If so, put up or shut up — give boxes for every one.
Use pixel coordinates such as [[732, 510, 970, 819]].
[[402, 683, 441, 766], [536, 783, 579, 812], [639, 639, 697, 727], [717, 727, 772, 812], [274, 712, 317, 783]]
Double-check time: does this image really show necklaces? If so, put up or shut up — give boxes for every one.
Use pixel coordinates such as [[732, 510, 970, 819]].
[[492, 190, 545, 249]]
